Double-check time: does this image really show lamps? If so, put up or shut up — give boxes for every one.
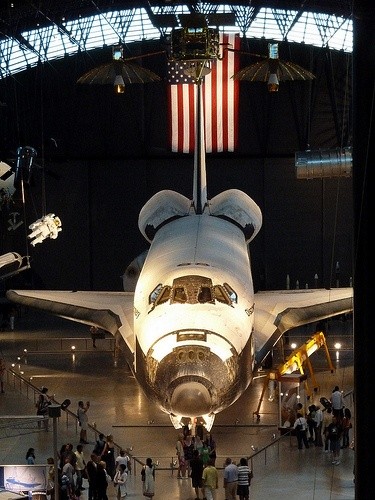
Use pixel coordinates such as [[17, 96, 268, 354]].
[[231, 43, 317, 92], [77, 43, 161, 96]]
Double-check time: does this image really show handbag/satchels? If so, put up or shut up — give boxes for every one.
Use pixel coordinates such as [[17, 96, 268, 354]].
[[83, 467, 89, 479], [113, 472, 118, 487], [296, 419, 303, 429], [141, 466, 146, 482]]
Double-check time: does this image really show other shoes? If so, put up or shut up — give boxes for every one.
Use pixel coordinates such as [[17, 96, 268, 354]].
[[79, 439, 83, 443], [177, 475, 181, 477], [82, 440, 89, 444], [78, 486, 85, 490], [336, 460, 340, 466], [182, 475, 186, 477], [332, 460, 336, 463]]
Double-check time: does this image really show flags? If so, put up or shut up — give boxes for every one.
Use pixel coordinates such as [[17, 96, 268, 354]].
[[163, 26, 241, 154]]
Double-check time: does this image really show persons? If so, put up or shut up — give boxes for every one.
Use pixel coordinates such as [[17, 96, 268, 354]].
[[288, 384, 352, 465], [114, 449, 132, 483], [77, 400, 90, 444], [141, 458, 157, 500], [35, 394, 48, 426], [113, 464, 128, 500], [25, 447, 36, 465], [39, 388, 52, 410], [43, 430, 119, 500], [175, 422, 254, 500]]
